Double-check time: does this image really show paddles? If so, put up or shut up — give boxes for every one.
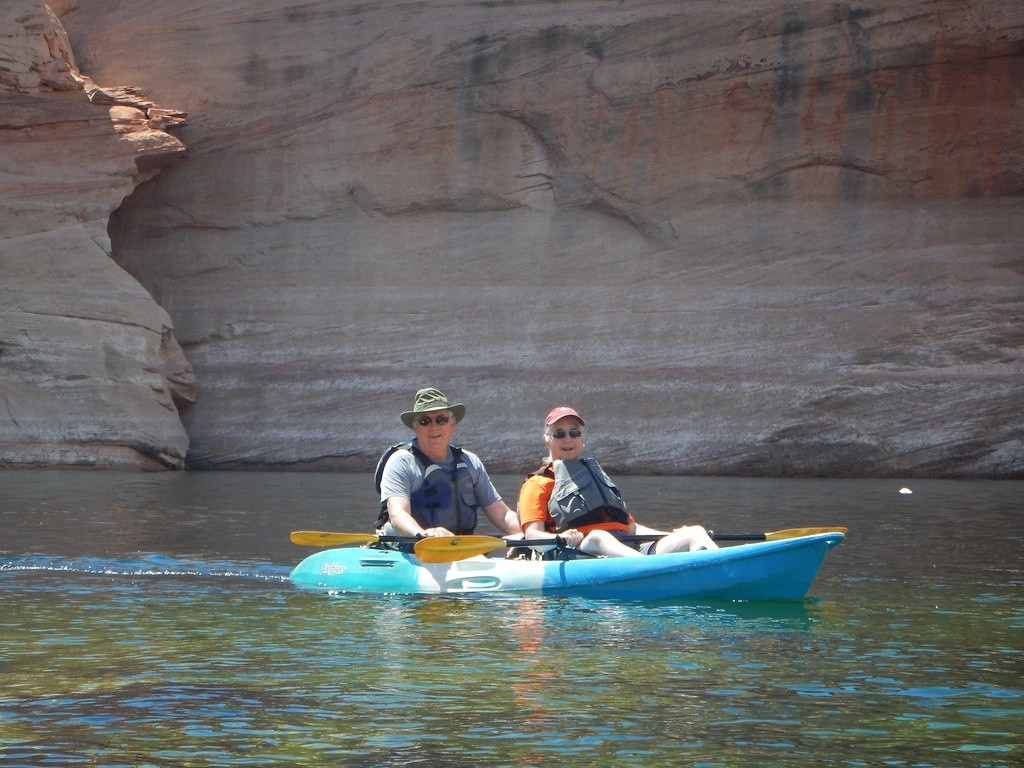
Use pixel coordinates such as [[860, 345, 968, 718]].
[[289, 529, 508, 548], [413, 525, 848, 566]]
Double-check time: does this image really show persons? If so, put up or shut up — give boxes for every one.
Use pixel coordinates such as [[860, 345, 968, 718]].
[[365, 387, 544, 561], [517, 405, 719, 560]]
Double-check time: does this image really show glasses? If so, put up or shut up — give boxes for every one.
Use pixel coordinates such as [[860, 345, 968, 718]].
[[416, 414, 455, 427], [548, 428, 583, 438]]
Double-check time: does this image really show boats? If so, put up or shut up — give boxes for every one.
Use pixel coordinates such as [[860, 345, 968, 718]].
[[288, 531, 846, 605]]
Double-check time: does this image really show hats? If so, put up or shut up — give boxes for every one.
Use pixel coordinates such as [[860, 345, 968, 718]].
[[400, 388, 465, 430], [545, 407, 585, 428]]
